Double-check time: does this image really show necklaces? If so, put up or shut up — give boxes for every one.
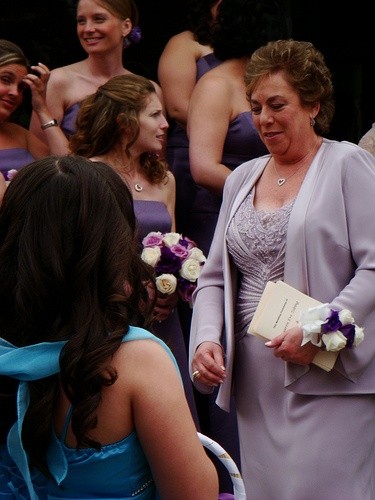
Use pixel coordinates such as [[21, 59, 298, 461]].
[[114, 158, 145, 192], [271, 155, 313, 185]]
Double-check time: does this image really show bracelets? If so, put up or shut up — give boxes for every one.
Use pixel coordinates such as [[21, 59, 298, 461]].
[[40, 117, 58, 130]]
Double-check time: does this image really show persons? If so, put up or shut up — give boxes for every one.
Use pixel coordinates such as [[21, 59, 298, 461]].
[[0, 0, 375, 500]]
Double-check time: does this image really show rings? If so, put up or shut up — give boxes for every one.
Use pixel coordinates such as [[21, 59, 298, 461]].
[[191, 370, 200, 378]]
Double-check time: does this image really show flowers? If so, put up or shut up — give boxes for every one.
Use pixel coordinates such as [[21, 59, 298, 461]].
[[145, 231, 208, 303], [7, 168, 17, 179]]
